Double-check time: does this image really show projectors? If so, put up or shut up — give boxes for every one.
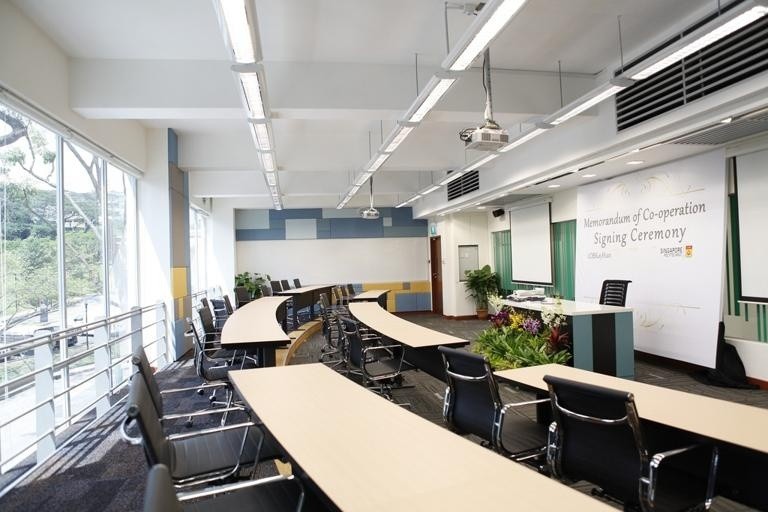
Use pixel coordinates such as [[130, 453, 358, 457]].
[[361, 211, 379, 219], [465, 128, 508, 153]]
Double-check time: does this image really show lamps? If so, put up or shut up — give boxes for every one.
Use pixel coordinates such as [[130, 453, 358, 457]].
[[393, 0, 767, 207], [337, 0, 530, 210], [210, 0, 283, 211]]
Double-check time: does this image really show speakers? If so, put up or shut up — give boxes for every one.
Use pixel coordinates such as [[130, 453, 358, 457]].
[[493, 208, 505, 217]]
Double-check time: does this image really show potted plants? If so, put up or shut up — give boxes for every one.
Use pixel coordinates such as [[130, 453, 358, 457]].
[[459, 264, 498, 320]]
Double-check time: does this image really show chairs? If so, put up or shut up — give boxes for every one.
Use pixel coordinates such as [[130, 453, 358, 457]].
[[184, 277, 417, 412], [599, 279, 632, 307], [119, 345, 723, 512]]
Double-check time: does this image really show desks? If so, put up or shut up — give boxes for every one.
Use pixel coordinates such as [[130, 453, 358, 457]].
[[490, 296, 635, 382], [348, 302, 470, 351], [491, 361, 767, 454]]
